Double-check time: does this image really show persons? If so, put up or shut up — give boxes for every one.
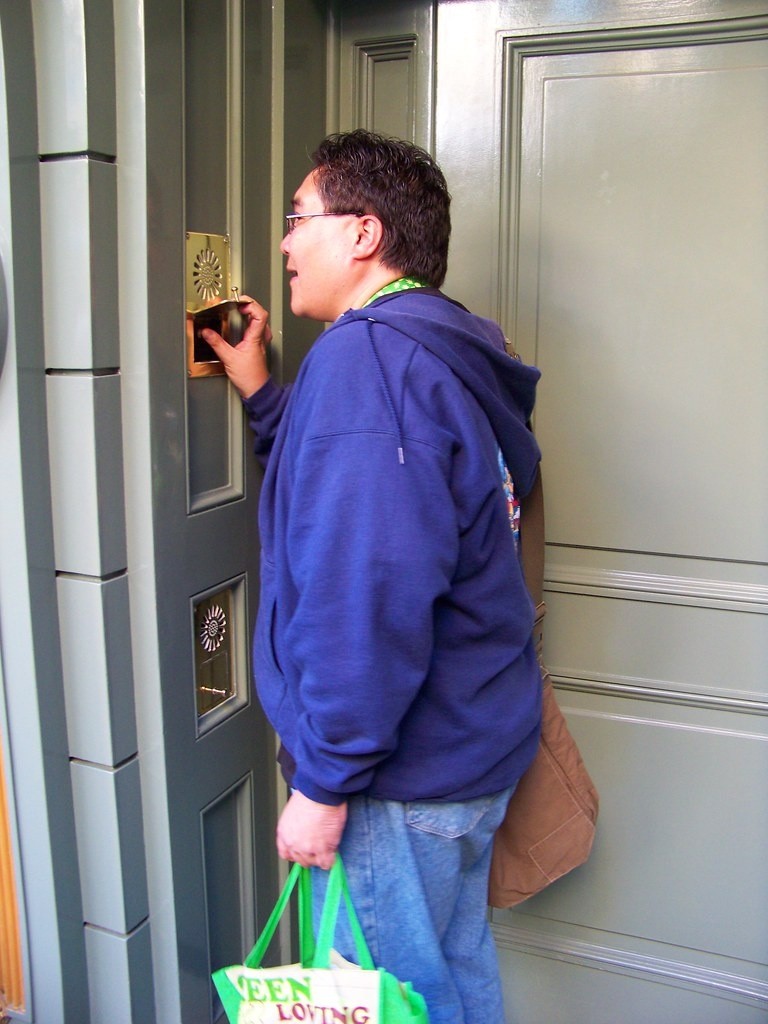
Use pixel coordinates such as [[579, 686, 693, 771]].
[[203, 126, 542, 1024]]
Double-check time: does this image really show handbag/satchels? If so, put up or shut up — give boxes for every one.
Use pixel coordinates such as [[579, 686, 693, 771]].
[[210, 852, 430, 1024], [487, 659, 599, 909]]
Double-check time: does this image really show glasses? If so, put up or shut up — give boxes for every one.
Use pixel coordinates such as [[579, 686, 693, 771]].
[[286, 210, 365, 235]]
